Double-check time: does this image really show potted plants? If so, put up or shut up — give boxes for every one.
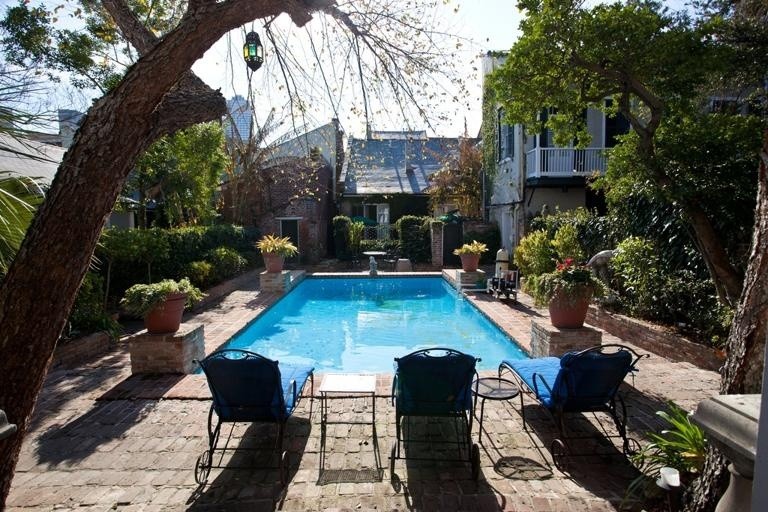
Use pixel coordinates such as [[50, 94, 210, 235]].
[[119, 276, 210, 334], [251, 233, 300, 272], [452, 240, 490, 273]]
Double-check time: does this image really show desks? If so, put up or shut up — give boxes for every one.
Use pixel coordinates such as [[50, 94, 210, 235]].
[[469, 376, 527, 444], [362, 250, 387, 269], [319, 373, 384, 482]]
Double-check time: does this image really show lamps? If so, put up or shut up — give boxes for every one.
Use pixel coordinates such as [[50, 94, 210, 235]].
[[243, 20, 263, 72]]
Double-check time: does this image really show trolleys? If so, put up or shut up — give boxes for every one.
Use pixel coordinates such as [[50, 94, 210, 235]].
[[488, 268, 518, 301]]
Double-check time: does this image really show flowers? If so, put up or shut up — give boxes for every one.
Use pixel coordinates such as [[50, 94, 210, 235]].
[[537, 257, 606, 310]]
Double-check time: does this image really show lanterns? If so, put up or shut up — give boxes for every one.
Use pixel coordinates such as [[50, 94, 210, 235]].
[[244, 32, 264, 71]]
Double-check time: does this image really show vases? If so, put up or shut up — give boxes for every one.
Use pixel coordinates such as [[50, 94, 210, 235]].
[[549, 284, 593, 328]]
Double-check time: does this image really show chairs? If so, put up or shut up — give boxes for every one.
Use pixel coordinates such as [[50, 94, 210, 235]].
[[387, 347, 483, 479], [383, 245, 402, 272], [196, 344, 316, 487], [496, 344, 644, 479]]
